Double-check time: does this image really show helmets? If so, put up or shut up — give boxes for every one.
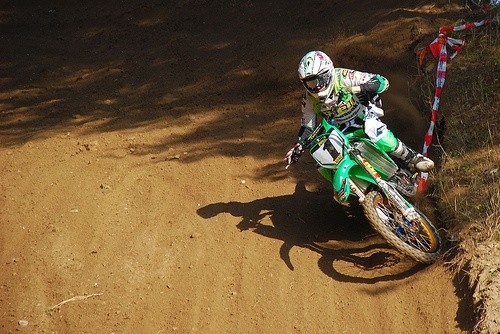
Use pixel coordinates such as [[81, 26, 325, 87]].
[[298, 51, 336, 102]]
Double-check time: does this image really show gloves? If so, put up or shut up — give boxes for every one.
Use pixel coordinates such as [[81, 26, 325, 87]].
[[284, 143, 303, 165], [338, 87, 353, 102]]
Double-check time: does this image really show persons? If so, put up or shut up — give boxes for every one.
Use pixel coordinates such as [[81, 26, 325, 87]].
[[284, 50, 434, 197]]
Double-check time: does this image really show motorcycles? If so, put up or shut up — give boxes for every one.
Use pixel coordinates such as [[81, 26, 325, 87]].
[[285, 88, 443, 265]]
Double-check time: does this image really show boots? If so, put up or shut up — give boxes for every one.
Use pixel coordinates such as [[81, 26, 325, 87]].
[[391, 139, 435, 171]]
